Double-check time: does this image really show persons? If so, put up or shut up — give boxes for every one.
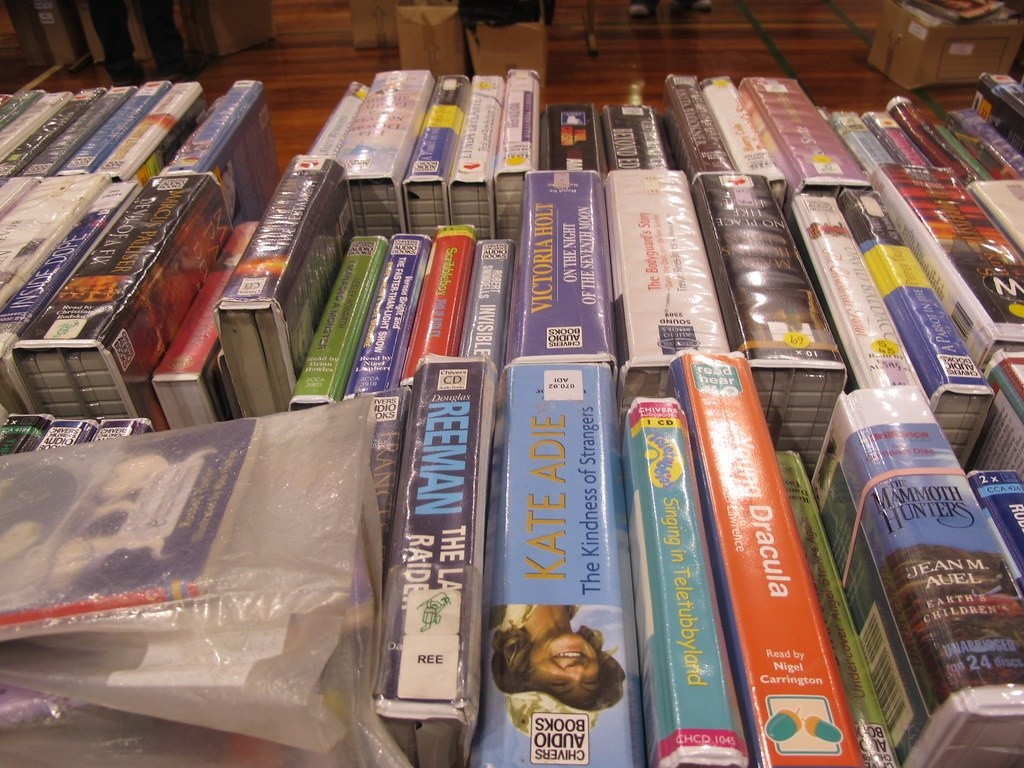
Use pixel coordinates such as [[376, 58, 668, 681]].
[[0, 70, 1024, 768], [495, 601, 624, 737], [90, 0, 213, 85]]
